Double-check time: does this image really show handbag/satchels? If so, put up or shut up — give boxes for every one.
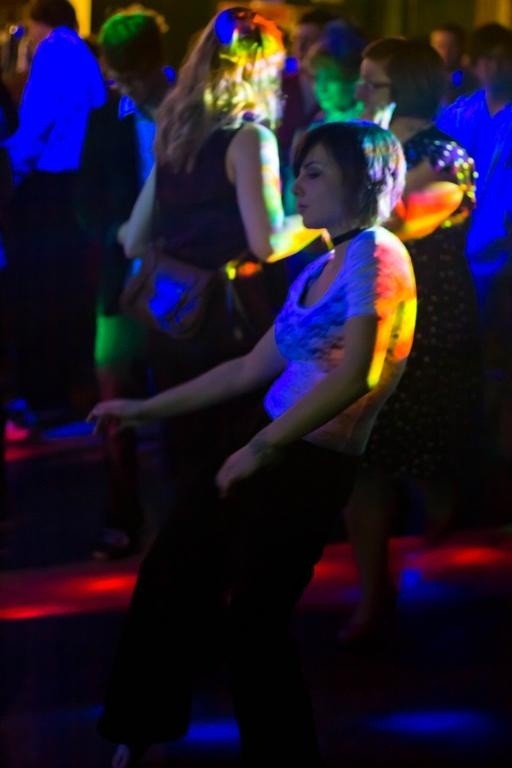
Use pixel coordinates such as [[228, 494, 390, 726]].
[[119, 244, 209, 342]]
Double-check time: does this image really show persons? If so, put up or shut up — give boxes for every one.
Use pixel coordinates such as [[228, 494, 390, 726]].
[[1, 1, 512, 768]]
